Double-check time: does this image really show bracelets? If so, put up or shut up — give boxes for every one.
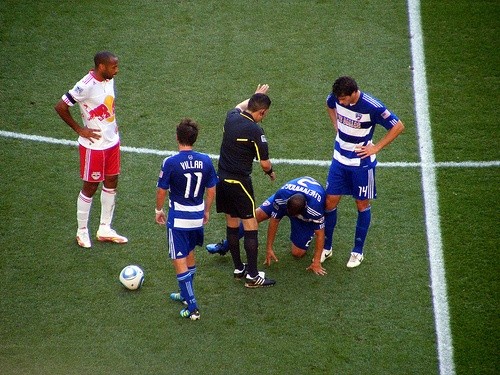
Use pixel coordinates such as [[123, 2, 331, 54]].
[[265, 168, 272, 174], [155, 208, 163, 214]]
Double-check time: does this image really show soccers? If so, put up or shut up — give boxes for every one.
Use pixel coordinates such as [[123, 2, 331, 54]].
[[119, 264, 145, 292]]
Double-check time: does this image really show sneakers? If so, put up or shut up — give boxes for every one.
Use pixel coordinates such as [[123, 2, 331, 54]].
[[180, 308, 200, 320], [347, 252, 363, 268], [312, 247, 332, 263], [206, 239, 228, 256], [234, 263, 249, 280], [170, 293, 187, 304], [245, 275, 276, 288]]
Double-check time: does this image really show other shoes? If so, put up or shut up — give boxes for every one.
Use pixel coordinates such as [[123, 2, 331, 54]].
[[96, 230, 128, 243], [76, 234, 91, 248]]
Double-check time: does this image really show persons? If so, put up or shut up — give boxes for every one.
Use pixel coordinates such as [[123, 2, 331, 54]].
[[206, 176, 328, 275], [54, 51, 128, 248], [154, 117, 219, 320], [216, 83, 276, 288], [312, 76, 404, 268]]
